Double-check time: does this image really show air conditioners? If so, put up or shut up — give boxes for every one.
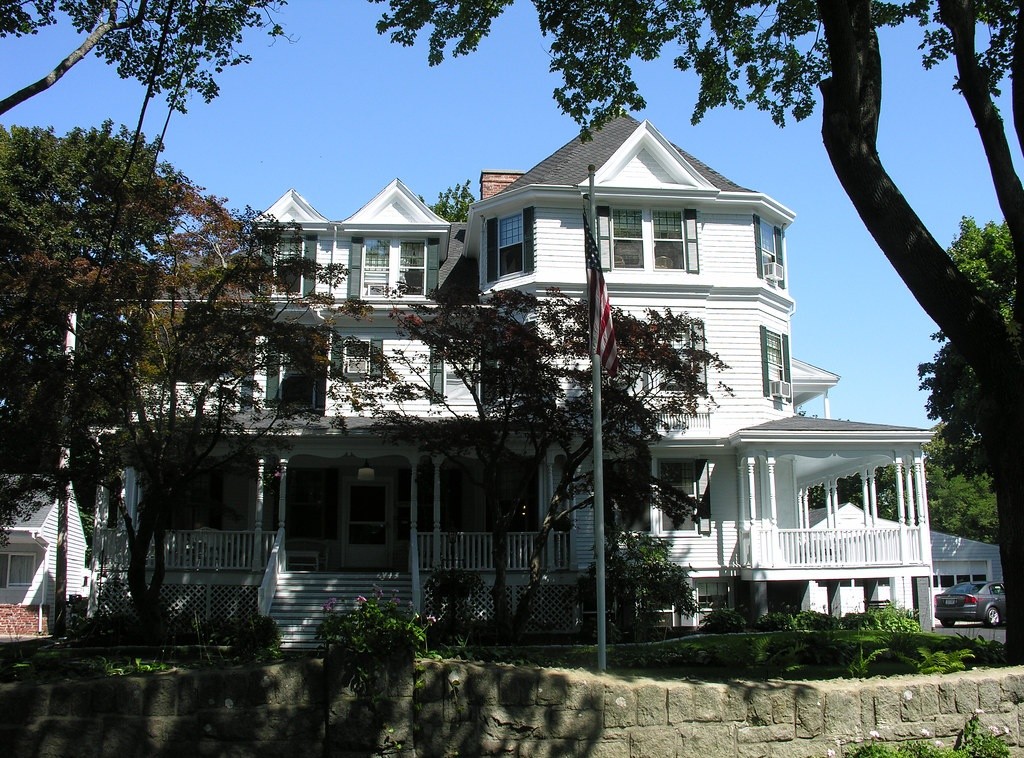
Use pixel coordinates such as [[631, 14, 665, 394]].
[[347, 358, 368, 374], [767, 262, 783, 281], [772, 380, 791, 398]]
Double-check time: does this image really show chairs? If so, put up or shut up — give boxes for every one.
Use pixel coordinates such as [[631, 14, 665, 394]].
[[406, 536, 433, 566], [193, 526, 223, 567]]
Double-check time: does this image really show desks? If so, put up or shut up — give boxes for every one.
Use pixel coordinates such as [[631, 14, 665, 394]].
[[286, 550, 319, 572]]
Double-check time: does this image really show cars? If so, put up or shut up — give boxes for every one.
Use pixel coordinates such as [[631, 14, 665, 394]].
[[934, 580, 1007, 628]]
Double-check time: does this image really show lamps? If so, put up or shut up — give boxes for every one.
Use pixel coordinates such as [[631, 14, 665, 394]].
[[357, 457, 375, 481]]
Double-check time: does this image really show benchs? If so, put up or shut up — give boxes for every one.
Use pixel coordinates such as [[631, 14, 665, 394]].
[[285, 539, 331, 572]]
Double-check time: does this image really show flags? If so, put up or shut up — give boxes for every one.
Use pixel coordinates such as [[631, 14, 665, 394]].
[[583, 206, 619, 377]]
